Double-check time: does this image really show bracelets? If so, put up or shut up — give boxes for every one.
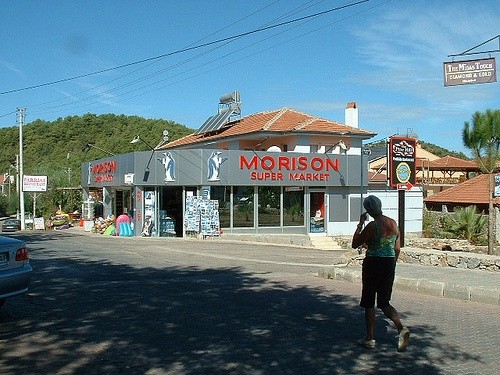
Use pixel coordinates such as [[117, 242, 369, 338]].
[[357, 224, 362, 228]]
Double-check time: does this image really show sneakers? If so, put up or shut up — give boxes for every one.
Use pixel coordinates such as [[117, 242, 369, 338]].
[[357, 336, 375, 348], [397, 325, 410, 353]]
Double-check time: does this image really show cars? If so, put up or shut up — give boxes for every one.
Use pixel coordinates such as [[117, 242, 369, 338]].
[[0, 236, 32, 307], [2, 219, 21, 232]]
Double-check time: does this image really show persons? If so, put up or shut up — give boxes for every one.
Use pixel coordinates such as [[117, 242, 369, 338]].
[[352, 195, 410, 351]]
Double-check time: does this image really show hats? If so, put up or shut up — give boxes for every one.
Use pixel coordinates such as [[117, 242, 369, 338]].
[[363, 194, 381, 211]]
